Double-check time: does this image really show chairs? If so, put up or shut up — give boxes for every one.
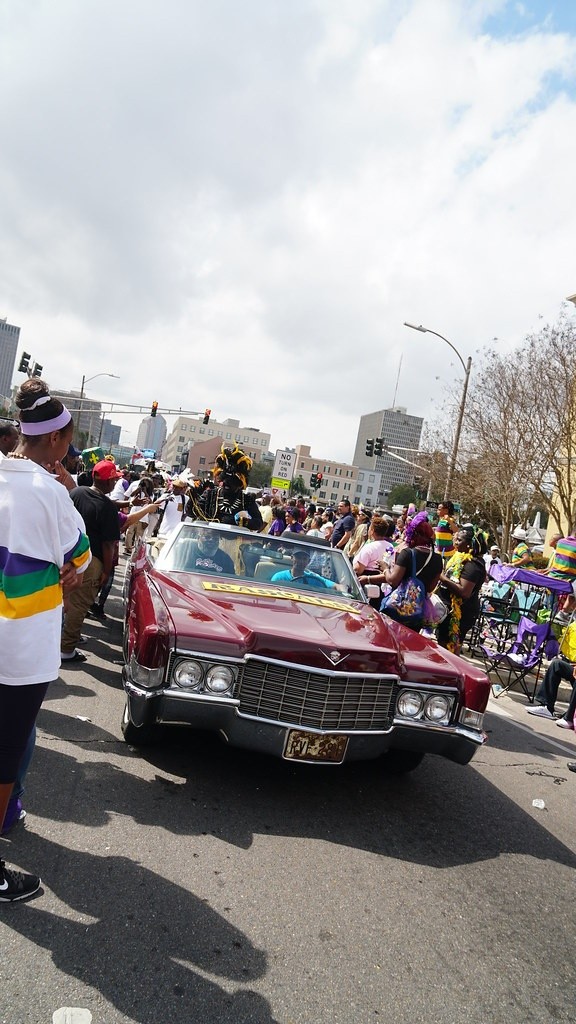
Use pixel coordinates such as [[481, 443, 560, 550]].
[[463, 564, 575, 704]]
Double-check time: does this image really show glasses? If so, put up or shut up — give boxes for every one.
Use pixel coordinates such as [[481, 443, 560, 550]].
[[199, 534, 219, 542]]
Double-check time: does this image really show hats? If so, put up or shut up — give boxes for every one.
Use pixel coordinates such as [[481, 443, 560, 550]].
[[510, 529, 527, 541], [292, 547, 310, 557], [92, 460, 124, 479], [491, 546, 501, 552], [172, 480, 184, 487]]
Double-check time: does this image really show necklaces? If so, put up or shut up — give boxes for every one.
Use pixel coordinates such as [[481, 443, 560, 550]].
[[9, 452, 27, 459]]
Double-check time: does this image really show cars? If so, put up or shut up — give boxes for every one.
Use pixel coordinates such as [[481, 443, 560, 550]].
[[113, 522, 492, 774]]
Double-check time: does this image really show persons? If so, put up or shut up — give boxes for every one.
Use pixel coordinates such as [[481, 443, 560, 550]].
[[0, 379, 93, 901], [538, 521, 576, 608], [525, 620, 576, 729], [0, 421, 19, 456], [60, 443, 485, 662], [483, 546, 502, 573], [503, 529, 536, 570]]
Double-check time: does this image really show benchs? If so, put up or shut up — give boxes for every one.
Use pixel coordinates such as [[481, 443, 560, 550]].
[[169, 538, 199, 568], [253, 555, 293, 580]]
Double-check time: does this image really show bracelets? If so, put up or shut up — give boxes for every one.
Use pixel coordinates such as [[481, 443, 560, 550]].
[[512, 563, 514, 567], [383, 569, 389, 575], [368, 575, 370, 583]]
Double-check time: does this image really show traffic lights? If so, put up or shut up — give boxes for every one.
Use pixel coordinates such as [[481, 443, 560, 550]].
[[366, 439, 374, 457], [310, 473, 322, 488], [203, 408, 212, 424], [18, 351, 31, 373], [32, 363, 43, 377], [151, 401, 158, 417], [374, 437, 385, 456]]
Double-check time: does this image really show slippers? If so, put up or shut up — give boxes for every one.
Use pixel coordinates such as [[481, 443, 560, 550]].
[[61, 650, 87, 661]]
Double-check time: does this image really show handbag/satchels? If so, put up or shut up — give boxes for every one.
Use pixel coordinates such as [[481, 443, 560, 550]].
[[379, 548, 424, 627], [422, 592, 448, 625]]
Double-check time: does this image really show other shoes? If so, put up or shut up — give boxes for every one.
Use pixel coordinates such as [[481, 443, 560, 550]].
[[94, 610, 107, 618], [556, 717, 574, 729], [525, 704, 553, 718], [89, 603, 98, 612], [567, 762, 576, 771], [77, 636, 88, 643]]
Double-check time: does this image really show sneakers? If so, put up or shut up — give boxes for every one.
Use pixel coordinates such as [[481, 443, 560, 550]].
[[0, 856, 41, 902]]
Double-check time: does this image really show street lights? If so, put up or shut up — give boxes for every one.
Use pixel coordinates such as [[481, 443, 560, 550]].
[[75, 373, 120, 448], [404, 322, 472, 499]]
